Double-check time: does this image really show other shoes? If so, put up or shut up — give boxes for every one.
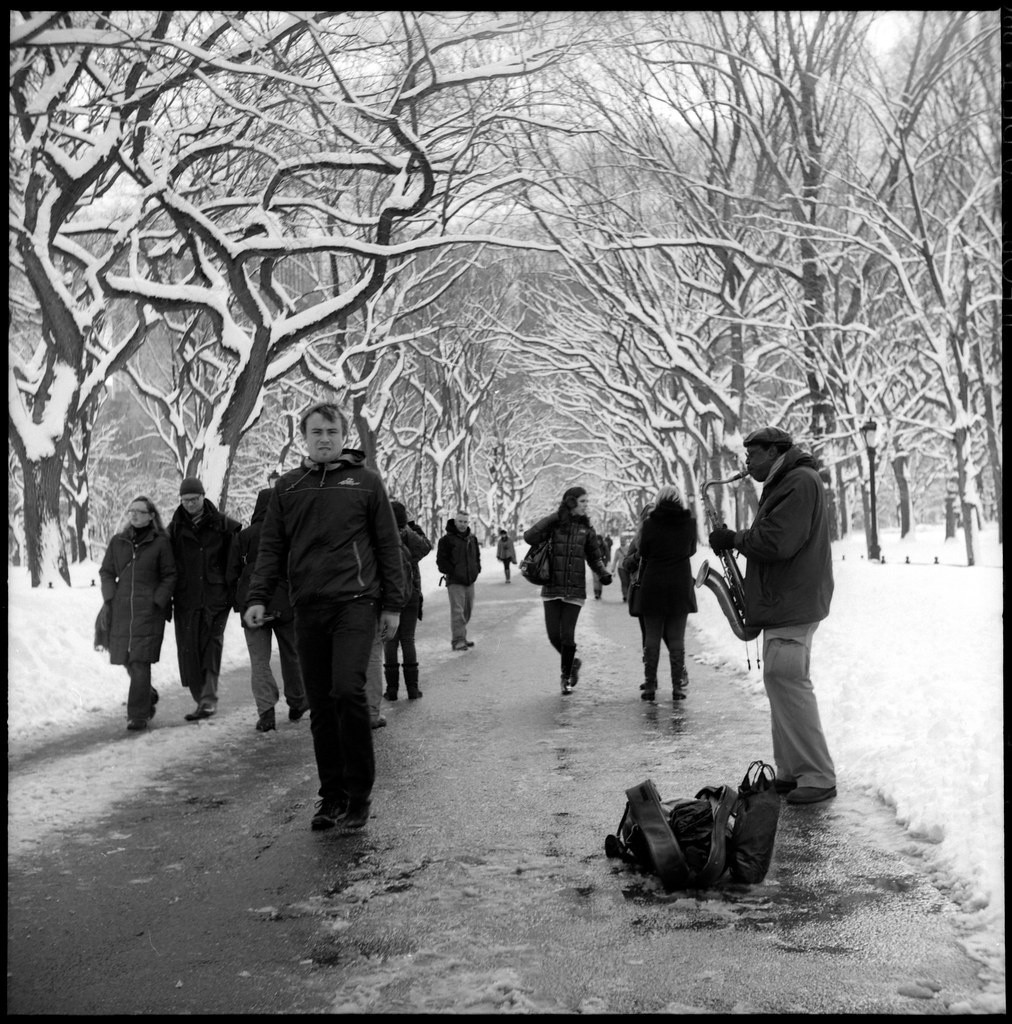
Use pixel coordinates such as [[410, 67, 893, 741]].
[[371, 715, 387, 728], [124, 682, 309, 735], [452, 639, 474, 650], [639, 683, 656, 701], [775, 779, 835, 803], [673, 678, 689, 700], [569, 659, 581, 687], [561, 679, 572, 694]]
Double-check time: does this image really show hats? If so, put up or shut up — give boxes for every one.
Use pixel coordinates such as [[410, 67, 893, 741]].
[[744, 427, 791, 447], [390, 501, 408, 527], [180, 477, 205, 496]]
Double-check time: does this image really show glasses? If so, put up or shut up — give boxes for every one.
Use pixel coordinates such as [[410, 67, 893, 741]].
[[745, 448, 762, 459], [128, 510, 149, 516]]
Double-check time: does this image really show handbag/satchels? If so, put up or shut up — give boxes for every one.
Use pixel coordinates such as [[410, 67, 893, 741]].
[[520, 538, 552, 585], [731, 760, 778, 884], [628, 580, 645, 616], [94, 602, 112, 652]]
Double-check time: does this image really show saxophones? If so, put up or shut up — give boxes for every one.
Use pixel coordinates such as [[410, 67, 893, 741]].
[[695, 469, 762, 641]]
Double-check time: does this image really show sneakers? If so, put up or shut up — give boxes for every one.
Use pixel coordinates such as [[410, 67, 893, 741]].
[[313, 798, 347, 830], [344, 798, 371, 830]]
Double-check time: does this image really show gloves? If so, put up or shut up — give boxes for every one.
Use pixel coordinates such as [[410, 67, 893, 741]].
[[599, 574, 612, 585], [709, 524, 736, 556], [558, 508, 568, 519]]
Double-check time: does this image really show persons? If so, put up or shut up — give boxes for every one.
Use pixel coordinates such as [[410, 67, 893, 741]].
[[625, 483, 698, 699], [525, 486, 613, 693], [496, 530, 518, 583], [713, 427, 835, 804], [99, 497, 175, 729], [228, 487, 431, 731], [593, 533, 629, 602], [437, 511, 481, 650], [239, 403, 403, 831], [167, 478, 242, 720]]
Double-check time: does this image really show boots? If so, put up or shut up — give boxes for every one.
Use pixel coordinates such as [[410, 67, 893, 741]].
[[383, 663, 399, 701], [402, 662, 422, 699]]
